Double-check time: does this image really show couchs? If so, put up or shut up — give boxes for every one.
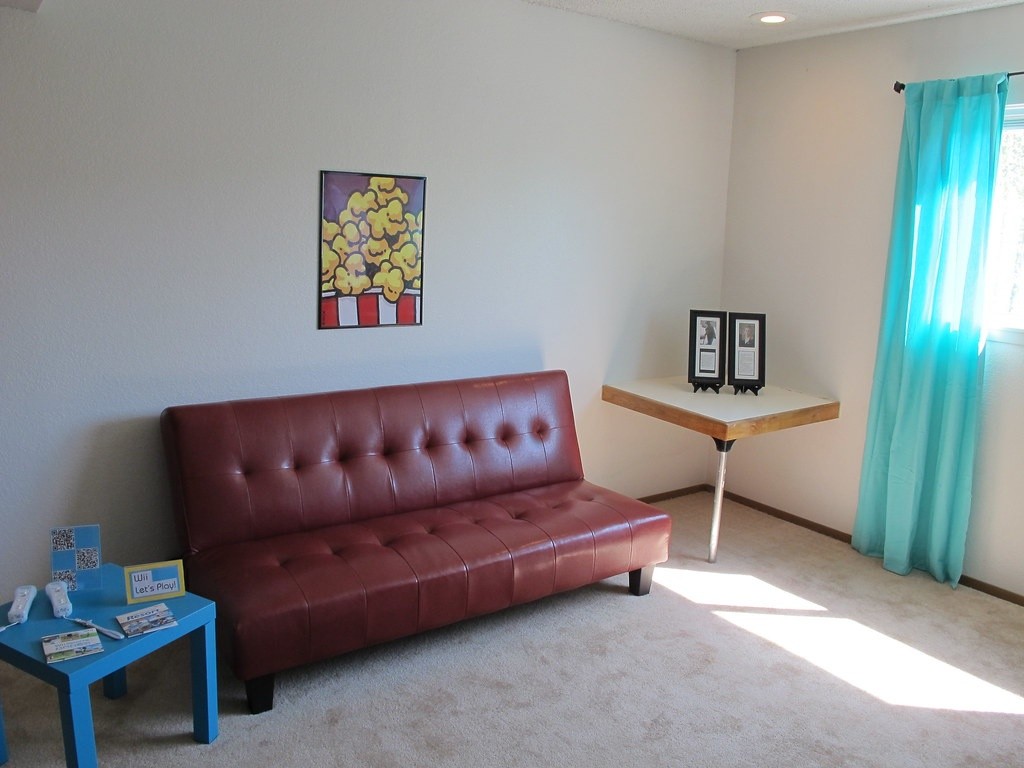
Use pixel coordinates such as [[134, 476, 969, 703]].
[[160, 369, 672, 713]]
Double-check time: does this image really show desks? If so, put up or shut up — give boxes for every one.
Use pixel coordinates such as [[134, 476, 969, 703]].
[[602, 370, 842, 564], [0, 562, 220, 768]]
